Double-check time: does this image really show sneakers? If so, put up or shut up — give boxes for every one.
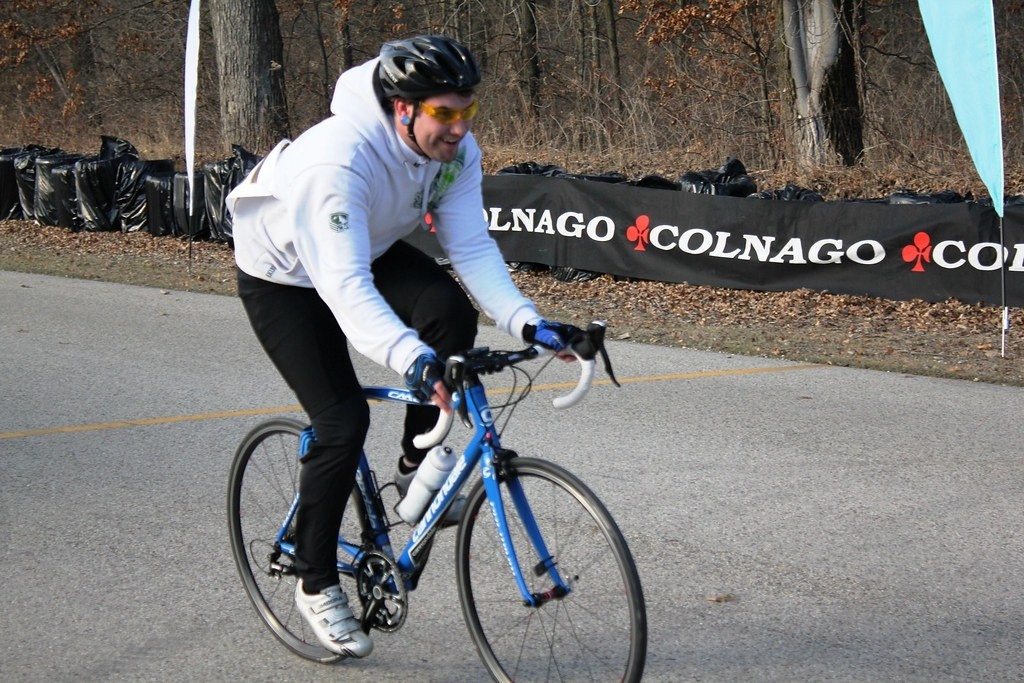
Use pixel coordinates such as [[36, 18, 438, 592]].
[[294, 578, 372, 657], [394, 461, 469, 522]]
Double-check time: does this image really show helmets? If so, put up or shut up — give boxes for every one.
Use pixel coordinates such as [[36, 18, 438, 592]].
[[378, 35, 482, 100]]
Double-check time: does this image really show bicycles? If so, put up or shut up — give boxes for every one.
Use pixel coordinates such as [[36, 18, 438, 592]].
[[225, 318, 650, 683]]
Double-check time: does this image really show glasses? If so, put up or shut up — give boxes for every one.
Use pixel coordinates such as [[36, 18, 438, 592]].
[[419, 100, 478, 124]]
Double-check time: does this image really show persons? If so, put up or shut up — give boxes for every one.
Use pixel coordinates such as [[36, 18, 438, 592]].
[[224, 35, 577, 660]]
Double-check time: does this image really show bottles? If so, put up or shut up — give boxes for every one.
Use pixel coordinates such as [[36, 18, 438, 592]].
[[398, 445, 457, 524]]
